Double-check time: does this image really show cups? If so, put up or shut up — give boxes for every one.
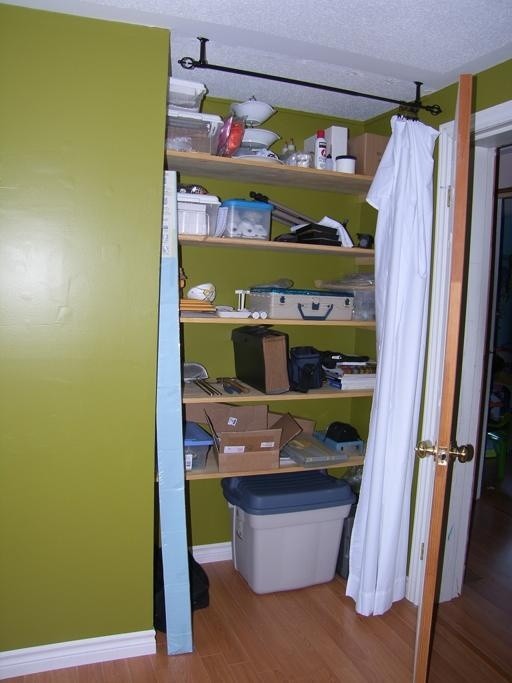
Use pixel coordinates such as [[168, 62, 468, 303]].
[[335, 154, 357, 175]]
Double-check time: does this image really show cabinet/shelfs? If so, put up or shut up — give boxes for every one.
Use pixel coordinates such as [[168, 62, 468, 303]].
[[166, 149, 376, 484]]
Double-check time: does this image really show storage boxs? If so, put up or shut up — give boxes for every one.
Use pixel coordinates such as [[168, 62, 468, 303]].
[[168, 77, 207, 113], [353, 131, 390, 174], [184, 418, 213, 471], [248, 286, 354, 324], [169, 108, 223, 155], [175, 192, 220, 236], [214, 428, 282, 472], [222, 200, 275, 240], [325, 274, 378, 322], [230, 324, 291, 394], [221, 471, 356, 595]]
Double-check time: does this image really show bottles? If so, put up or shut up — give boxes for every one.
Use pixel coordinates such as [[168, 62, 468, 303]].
[[282, 129, 334, 172]]
